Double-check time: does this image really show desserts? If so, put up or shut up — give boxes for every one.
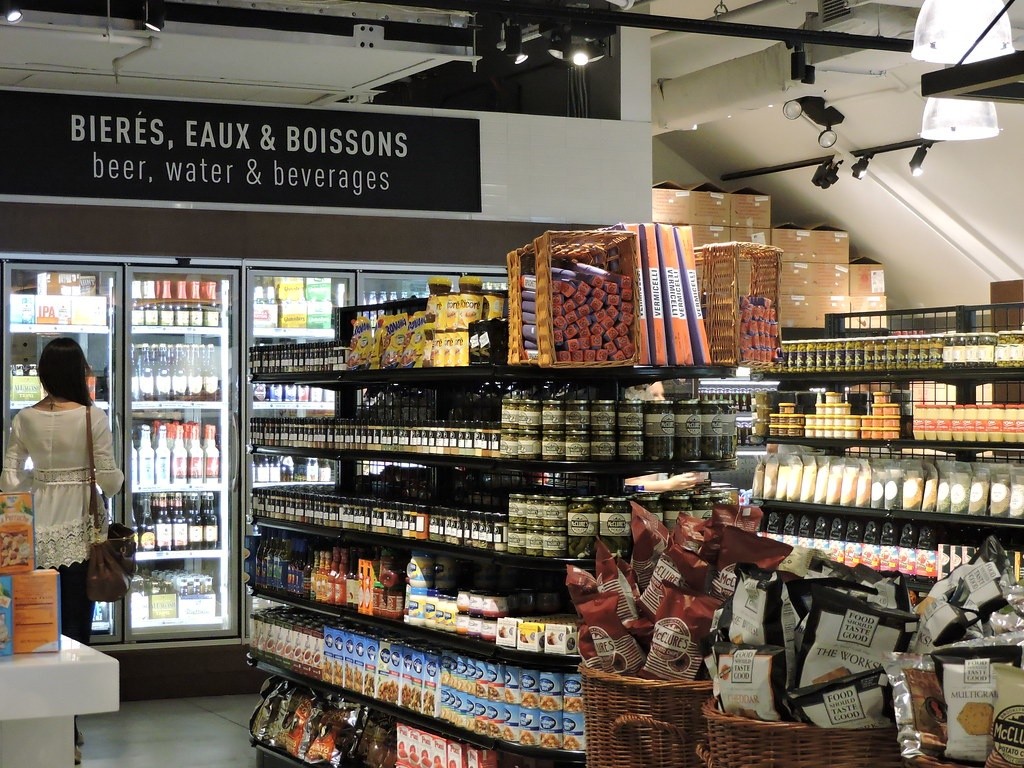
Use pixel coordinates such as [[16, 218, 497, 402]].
[[322, 659, 585, 750]]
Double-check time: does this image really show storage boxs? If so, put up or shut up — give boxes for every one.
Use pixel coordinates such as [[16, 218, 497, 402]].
[[992, 280, 1024, 457], [275, 276, 331, 329], [496, 615, 578, 655], [10, 273, 107, 326], [652, 181, 888, 329], [1, 493, 62, 657]]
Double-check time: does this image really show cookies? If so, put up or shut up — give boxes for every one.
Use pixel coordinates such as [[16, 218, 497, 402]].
[[957, 703, 995, 736], [812, 667, 852, 684]]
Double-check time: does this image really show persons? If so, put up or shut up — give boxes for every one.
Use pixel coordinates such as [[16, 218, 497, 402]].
[[0, 337, 124, 764]]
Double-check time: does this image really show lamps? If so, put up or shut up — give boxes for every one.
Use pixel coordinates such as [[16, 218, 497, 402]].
[[548, 18, 606, 66], [812, 160, 844, 188], [919, 97, 999, 142], [911, 0, 1014, 64], [0, 0, 23, 25], [852, 152, 874, 180], [785, 42, 816, 85], [505, 16, 528, 64], [910, 142, 933, 176], [144, 0, 164, 32], [783, 96, 844, 148]]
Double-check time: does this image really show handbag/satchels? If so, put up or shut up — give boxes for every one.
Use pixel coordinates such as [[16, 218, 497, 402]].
[[86, 522, 138, 601]]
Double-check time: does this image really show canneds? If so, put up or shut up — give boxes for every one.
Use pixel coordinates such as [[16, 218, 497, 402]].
[[249, 416, 501, 458], [401, 550, 561, 643], [768, 331, 1024, 444], [499, 397, 738, 461], [503, 483, 740, 559], [250, 486, 511, 553], [249, 340, 352, 374]]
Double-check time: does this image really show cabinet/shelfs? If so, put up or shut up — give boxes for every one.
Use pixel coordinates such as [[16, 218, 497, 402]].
[[246, 366, 741, 768], [748, 369, 1024, 594]]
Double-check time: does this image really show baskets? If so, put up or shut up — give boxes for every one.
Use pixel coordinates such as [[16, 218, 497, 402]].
[[578, 664, 714, 768], [904, 757, 976, 768], [505, 231, 642, 367], [695, 696, 909, 768], [701, 242, 784, 367]]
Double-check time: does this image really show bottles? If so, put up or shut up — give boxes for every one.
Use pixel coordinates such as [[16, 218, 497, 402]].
[[752, 391, 773, 436], [756, 509, 1021, 590], [11, 277, 509, 657], [913, 403, 1024, 443], [802, 391, 901, 440]]
